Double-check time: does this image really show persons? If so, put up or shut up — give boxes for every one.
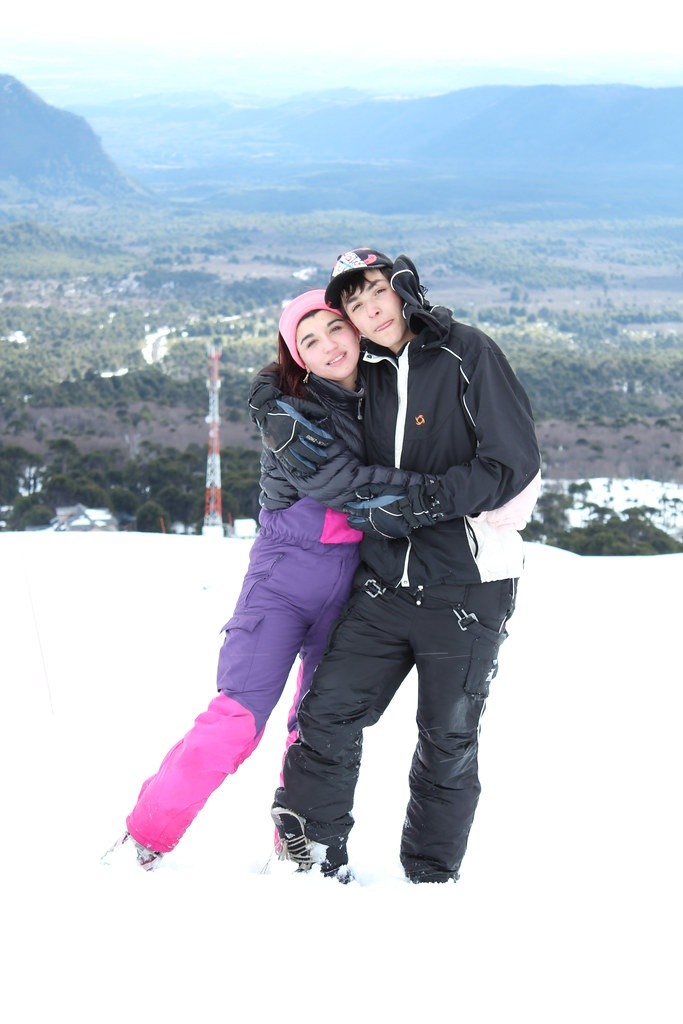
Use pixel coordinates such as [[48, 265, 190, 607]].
[[94, 246, 544, 891]]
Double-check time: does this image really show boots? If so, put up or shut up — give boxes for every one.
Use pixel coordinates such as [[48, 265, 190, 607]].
[[271, 807, 355, 884]]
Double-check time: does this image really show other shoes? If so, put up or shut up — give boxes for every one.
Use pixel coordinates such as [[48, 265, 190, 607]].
[[100, 831, 165, 873]]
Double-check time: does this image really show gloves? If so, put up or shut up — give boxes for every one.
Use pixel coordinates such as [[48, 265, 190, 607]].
[[248, 384, 334, 477], [344, 484, 436, 541]]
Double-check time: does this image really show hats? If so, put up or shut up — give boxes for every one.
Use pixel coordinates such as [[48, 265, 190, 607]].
[[325, 248, 394, 309]]
